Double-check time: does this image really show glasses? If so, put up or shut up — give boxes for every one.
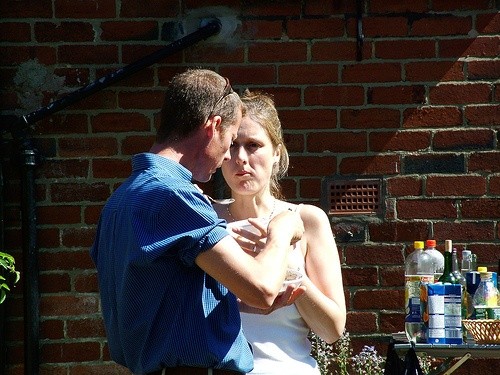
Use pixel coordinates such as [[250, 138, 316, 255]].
[[203, 77, 233, 128]]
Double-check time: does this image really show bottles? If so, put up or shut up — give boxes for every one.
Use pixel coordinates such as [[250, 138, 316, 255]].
[[461, 250, 471, 277], [439, 240, 459, 284], [469, 254, 477, 272], [405, 241, 434, 340], [472, 272, 500, 319], [424, 240, 444, 283], [453, 248, 467, 337]]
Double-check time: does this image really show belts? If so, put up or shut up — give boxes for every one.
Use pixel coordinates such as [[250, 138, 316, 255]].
[[147, 365, 242, 375]]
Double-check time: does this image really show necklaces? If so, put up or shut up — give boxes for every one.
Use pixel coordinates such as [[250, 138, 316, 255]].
[[226, 196, 275, 223]]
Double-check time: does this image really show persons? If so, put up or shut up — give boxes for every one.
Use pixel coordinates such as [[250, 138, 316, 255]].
[[89, 68, 305, 375], [212, 88, 346, 375]]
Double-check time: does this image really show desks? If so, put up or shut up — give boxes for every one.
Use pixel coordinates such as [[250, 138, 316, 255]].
[[393, 341, 500, 375]]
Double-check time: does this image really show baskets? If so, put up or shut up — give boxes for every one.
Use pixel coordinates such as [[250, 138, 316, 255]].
[[462, 318, 500, 345]]
